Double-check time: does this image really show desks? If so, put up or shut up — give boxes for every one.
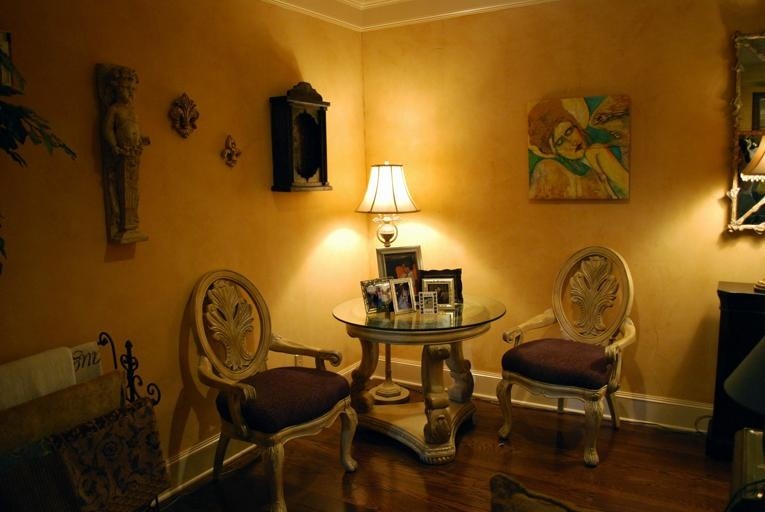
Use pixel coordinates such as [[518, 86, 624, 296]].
[[333, 298, 507, 464]]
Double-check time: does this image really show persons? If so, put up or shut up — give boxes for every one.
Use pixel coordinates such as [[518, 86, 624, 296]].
[[397, 283, 411, 309], [406, 266, 416, 288], [375, 287, 384, 307], [433, 286, 445, 304], [103, 64, 151, 158], [397, 263, 408, 278]]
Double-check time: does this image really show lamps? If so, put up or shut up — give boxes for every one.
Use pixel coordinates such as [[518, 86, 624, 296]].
[[354, 160, 422, 247], [740, 136, 765, 293]]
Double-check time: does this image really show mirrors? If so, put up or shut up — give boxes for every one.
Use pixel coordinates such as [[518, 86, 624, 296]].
[[725, 32, 765, 236]]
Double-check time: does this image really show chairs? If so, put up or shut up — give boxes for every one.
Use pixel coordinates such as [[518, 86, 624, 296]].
[[496, 245, 636, 466], [186, 270, 359, 512]]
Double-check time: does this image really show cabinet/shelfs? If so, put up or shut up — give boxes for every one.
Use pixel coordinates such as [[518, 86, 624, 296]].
[[707, 281, 765, 475]]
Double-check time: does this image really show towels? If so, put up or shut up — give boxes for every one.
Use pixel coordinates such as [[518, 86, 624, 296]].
[[0, 341, 170, 511]]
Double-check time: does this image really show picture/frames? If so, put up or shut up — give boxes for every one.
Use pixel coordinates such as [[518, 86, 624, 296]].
[[752, 91, 765, 130], [418, 292, 438, 314], [389, 278, 416, 315], [416, 269, 463, 304], [376, 244, 422, 305], [422, 278, 455, 309], [360, 276, 394, 315]]
